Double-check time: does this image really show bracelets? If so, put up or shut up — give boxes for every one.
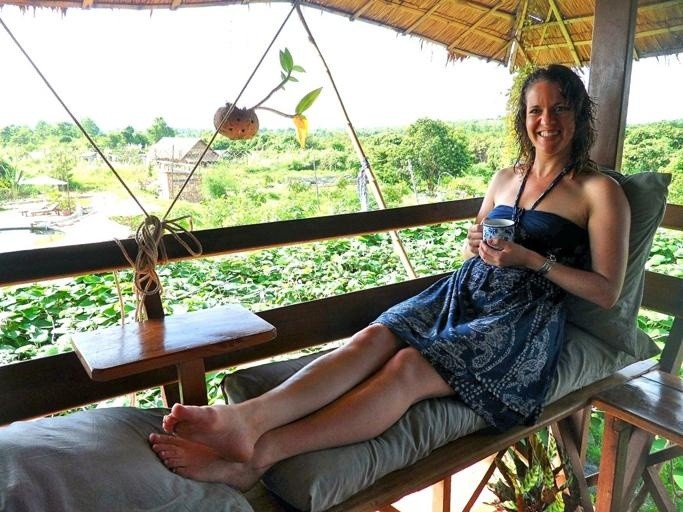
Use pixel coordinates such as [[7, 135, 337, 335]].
[[535, 254, 557, 276]]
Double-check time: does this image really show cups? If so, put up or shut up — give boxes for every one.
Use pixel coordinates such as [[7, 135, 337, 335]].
[[480, 218, 515, 251]]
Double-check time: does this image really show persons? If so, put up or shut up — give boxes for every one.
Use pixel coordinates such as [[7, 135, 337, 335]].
[[149, 65, 631, 493]]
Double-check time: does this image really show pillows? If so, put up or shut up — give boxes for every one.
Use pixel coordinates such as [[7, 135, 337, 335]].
[[225, 332, 661, 511], [564, 172, 671, 355], [0, 406, 253, 512]]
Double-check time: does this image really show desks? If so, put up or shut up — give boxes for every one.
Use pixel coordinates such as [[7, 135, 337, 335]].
[[70, 305, 277, 407]]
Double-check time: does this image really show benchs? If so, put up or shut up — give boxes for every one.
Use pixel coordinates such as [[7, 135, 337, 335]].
[[593, 368, 683, 509], [0, 196, 663, 510]]
